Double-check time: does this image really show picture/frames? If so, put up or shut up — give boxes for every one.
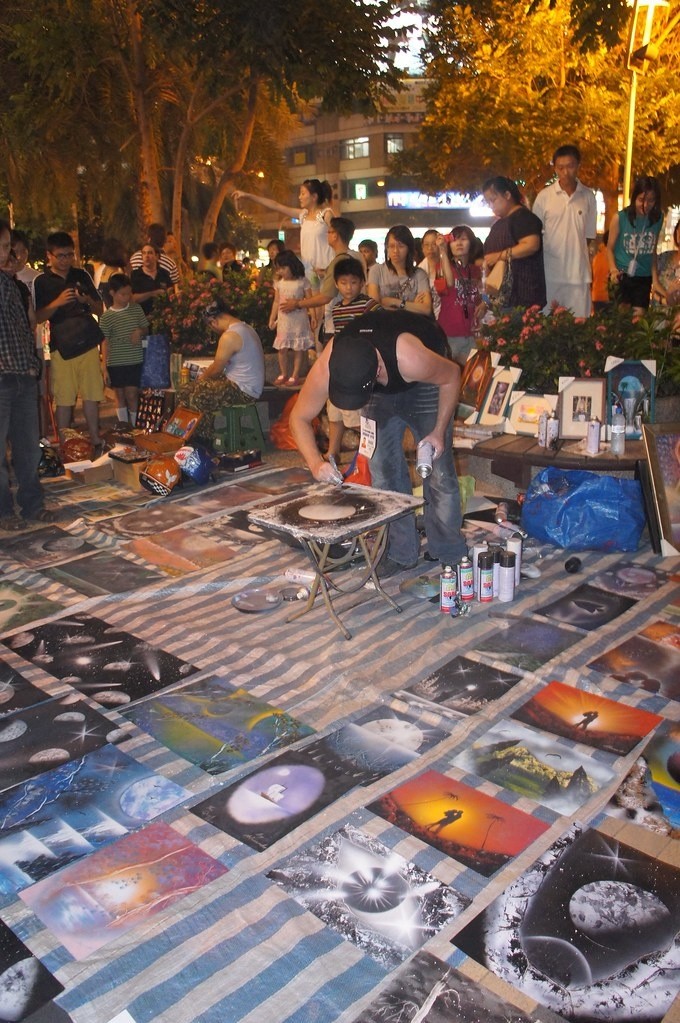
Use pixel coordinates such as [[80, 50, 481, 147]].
[[504, 391, 558, 438], [642, 423, 680, 557], [605, 356, 656, 440], [557, 378, 605, 439], [477, 365, 523, 425], [455, 349, 501, 425]]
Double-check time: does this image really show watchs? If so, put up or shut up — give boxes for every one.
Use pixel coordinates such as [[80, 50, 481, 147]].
[[400, 300, 405, 308]]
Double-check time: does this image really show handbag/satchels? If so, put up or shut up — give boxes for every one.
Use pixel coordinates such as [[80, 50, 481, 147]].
[[51, 318, 105, 360], [485, 247, 515, 306], [520, 466, 646, 554]]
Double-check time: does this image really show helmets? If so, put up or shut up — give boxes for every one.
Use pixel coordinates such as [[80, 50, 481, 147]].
[[138, 454, 184, 496], [173, 445, 216, 484], [60, 438, 95, 467], [33, 445, 65, 477]]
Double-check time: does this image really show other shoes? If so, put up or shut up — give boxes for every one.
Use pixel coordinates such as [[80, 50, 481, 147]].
[[0, 513, 27, 530], [27, 508, 58, 523], [369, 556, 418, 581]]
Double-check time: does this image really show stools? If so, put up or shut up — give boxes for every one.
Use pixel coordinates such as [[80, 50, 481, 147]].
[[212, 403, 266, 453]]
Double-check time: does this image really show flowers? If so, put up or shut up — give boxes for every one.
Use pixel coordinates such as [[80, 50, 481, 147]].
[[151, 260, 276, 355], [477, 272, 680, 398]]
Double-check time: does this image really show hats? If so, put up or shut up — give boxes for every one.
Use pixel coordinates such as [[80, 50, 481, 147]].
[[328, 336, 378, 411]]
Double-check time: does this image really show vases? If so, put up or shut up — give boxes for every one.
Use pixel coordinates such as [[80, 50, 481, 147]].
[[654, 398, 680, 423], [187, 352, 311, 382]]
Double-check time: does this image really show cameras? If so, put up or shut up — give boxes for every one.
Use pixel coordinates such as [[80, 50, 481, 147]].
[[628, 260, 637, 277], [71, 279, 90, 297]]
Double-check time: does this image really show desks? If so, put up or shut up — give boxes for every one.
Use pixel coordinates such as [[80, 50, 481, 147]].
[[247, 481, 429, 641]]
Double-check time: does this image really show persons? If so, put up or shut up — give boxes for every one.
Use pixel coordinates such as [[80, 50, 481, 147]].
[[290, 309, 469, 581], [0, 145, 680, 533]]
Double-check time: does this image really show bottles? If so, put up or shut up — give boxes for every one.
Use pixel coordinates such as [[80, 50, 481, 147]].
[[610, 408, 626, 455], [587, 415, 600, 455], [494, 503, 509, 525], [296, 578, 331, 601], [538, 409, 559, 450], [416, 441, 433, 479], [285, 568, 322, 582], [478, 264, 493, 308], [180, 362, 190, 384], [438, 532, 523, 615]]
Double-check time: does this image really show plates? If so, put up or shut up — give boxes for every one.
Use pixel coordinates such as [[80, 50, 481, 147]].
[[231, 589, 284, 612]]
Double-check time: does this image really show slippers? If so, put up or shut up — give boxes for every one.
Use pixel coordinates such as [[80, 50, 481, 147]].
[[274, 375, 289, 385], [285, 376, 305, 388]]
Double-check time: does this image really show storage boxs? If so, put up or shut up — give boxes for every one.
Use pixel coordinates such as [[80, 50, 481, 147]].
[[71, 464, 113, 483], [135, 406, 204, 452], [113, 393, 165, 446], [113, 458, 147, 490]]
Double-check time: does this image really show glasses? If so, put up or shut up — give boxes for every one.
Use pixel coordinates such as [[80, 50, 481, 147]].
[[49, 251, 74, 260]]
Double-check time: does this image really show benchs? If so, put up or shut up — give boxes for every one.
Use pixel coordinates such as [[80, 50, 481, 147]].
[[452, 432, 645, 489]]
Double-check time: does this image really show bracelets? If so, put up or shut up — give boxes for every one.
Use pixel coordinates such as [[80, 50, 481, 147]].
[[482, 300, 489, 308], [440, 253, 447, 259], [297, 298, 301, 310]]
